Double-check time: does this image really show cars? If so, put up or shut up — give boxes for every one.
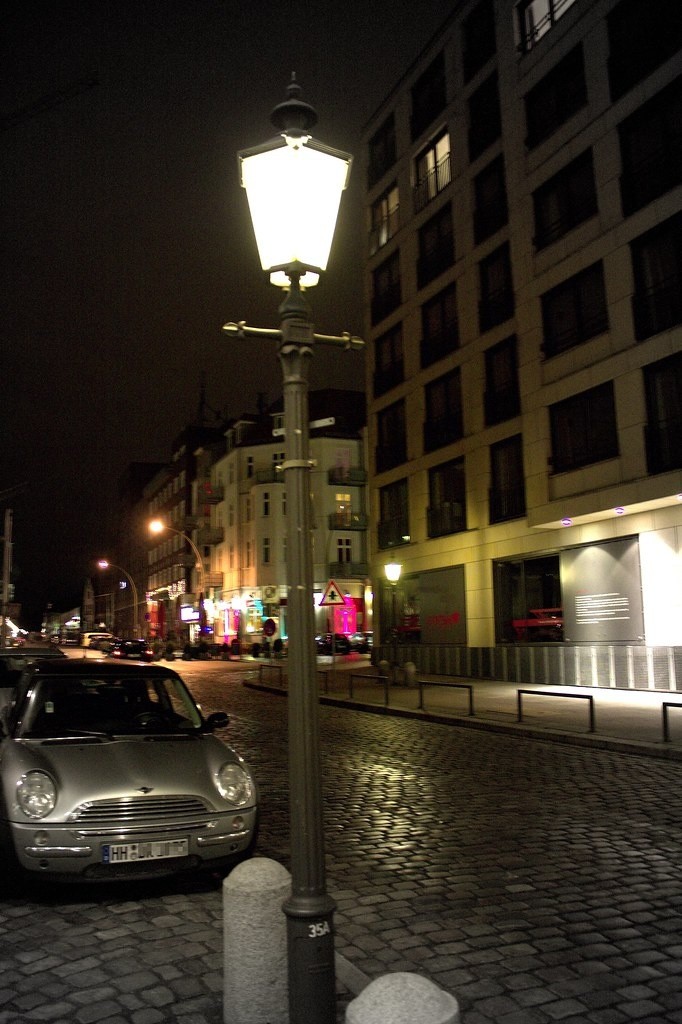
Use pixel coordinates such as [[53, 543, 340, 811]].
[[350, 631, 374, 646], [82, 630, 151, 658], [0, 631, 66, 673], [315, 633, 351, 653], [0, 659, 258, 882]]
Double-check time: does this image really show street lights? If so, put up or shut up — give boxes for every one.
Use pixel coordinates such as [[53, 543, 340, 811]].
[[98, 560, 138, 640], [384, 552, 403, 668], [150, 522, 207, 644], [221, 70, 365, 1024]]
